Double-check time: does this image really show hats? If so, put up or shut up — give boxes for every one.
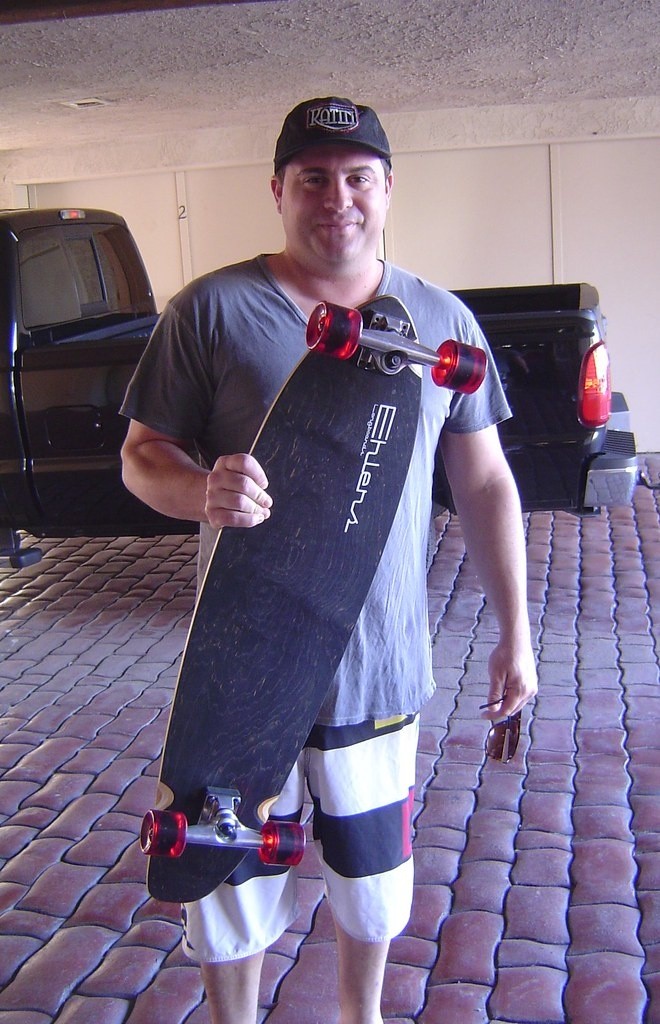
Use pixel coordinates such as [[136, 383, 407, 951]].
[[272, 97, 392, 176]]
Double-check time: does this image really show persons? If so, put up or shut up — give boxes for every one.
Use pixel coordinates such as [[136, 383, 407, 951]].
[[117, 97, 542, 1024]]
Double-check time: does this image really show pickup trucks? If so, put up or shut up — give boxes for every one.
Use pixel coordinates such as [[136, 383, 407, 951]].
[[0, 207, 639, 569]]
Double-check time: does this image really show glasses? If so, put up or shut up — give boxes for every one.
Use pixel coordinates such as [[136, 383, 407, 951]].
[[479, 692, 523, 764]]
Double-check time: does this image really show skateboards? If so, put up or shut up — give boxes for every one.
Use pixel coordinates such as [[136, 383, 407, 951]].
[[133, 300, 490, 906]]
